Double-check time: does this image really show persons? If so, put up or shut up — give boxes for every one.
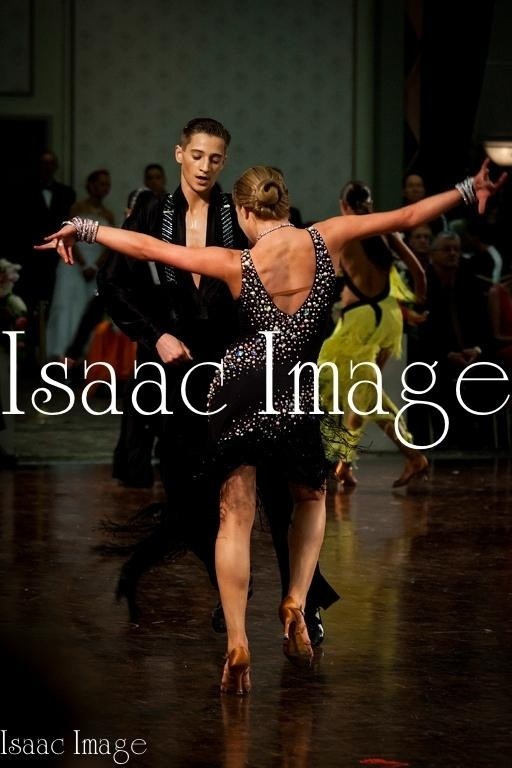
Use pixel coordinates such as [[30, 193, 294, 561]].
[[97, 118, 340, 648], [287, 172, 512, 493], [31, 155, 507, 698], [0, 149, 168, 395]]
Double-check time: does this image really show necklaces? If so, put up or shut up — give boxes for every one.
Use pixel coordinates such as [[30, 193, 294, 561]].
[[254, 224, 294, 241]]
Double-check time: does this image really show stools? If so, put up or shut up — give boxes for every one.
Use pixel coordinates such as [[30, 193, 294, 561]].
[[409, 387, 503, 488]]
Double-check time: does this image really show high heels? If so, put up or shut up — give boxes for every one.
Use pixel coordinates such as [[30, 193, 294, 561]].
[[392, 454, 430, 488], [278, 594, 314, 669], [220, 646, 251, 696], [336, 460, 356, 488]]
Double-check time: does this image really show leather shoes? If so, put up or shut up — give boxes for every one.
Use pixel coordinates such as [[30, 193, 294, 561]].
[[303, 606, 325, 646], [210, 596, 227, 633]]
